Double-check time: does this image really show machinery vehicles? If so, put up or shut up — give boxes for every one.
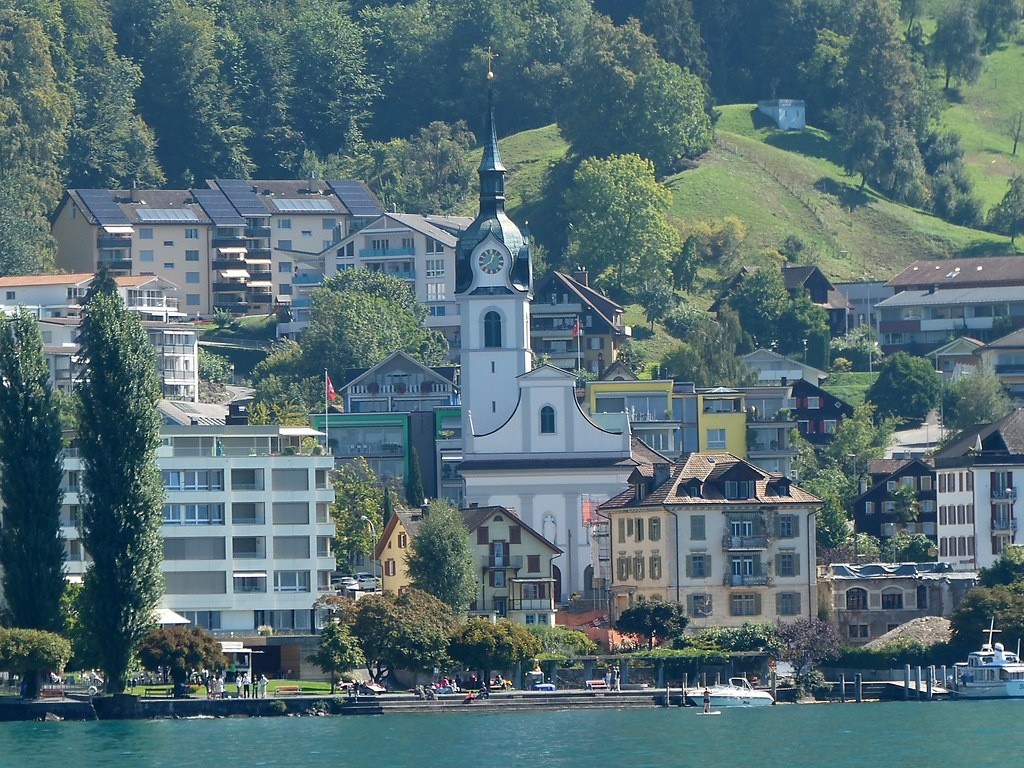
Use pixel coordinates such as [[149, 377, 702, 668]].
[[524, 659, 556, 691]]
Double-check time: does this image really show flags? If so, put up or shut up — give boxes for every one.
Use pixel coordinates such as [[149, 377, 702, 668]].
[[327, 374, 337, 400], [573, 320, 578, 336]]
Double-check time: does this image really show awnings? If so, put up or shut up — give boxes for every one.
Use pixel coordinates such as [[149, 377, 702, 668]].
[[232, 572, 267, 578]]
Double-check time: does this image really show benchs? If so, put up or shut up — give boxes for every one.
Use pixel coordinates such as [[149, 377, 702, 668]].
[[145, 688, 172, 697], [340, 683, 382, 697], [491, 680, 512, 689], [41, 689, 64, 701], [274, 686, 302, 697], [584, 680, 609, 690]]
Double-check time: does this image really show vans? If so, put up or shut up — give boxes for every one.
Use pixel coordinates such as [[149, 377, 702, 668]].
[[331, 577, 359, 591]]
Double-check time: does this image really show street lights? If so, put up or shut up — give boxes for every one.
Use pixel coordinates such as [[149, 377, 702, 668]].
[[847, 453, 855, 480], [361, 515, 376, 593], [921, 423, 928, 453], [935, 370, 943, 440]]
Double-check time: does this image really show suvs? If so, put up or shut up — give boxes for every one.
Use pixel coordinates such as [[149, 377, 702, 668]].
[[355, 573, 375, 592]]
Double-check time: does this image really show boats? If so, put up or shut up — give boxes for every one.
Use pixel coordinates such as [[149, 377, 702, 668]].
[[946, 614, 1024, 699], [686, 677, 775, 707]]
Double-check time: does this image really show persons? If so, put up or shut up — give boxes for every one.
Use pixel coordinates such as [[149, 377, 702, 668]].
[[251, 673, 258, 698], [260, 674, 268, 698], [243, 673, 250, 698], [751, 675, 760, 687], [81, 668, 90, 681], [196, 669, 226, 699], [377, 684, 384, 688], [605, 665, 621, 691], [230, 659, 238, 682], [409, 674, 503, 704], [354, 680, 360, 697], [236, 674, 242, 698], [90, 668, 96, 684], [368, 677, 374, 686], [702, 688, 711, 712]]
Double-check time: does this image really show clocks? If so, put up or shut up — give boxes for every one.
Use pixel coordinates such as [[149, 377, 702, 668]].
[[477, 248, 505, 275]]
